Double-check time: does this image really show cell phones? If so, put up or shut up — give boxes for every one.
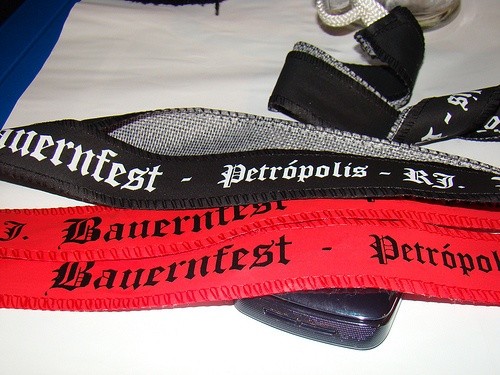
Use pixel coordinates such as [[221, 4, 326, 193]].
[[230, 288, 403, 351]]
